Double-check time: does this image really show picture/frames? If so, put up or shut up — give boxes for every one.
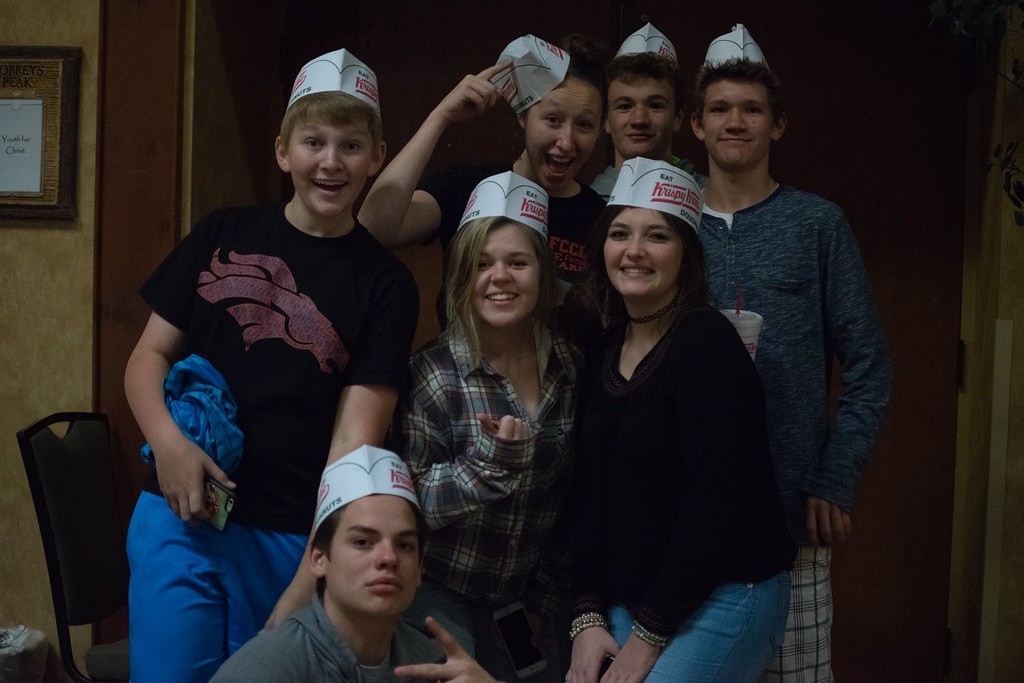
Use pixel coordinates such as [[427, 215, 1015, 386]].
[[0, 45, 82, 223]]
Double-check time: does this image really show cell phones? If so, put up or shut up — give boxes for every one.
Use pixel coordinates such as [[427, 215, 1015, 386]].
[[201, 476, 236, 532]]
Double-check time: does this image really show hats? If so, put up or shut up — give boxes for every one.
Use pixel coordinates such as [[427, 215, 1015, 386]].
[[615, 22, 679, 69], [310, 444, 424, 545], [286, 47, 380, 122], [458, 170, 551, 243], [703, 23, 769, 69], [607, 156, 704, 234], [488, 33, 572, 113]]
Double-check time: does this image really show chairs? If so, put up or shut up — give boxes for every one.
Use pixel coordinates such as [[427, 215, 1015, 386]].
[[18, 413, 132, 683]]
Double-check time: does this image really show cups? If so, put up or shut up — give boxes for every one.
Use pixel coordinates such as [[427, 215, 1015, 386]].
[[718, 309, 764, 365]]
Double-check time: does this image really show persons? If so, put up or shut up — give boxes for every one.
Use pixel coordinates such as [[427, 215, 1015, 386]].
[[120, 49, 419, 682], [562, 155, 797, 683], [383, 171, 589, 682], [353, 30, 610, 364], [585, 19, 708, 205], [206, 447, 506, 683], [689, 24, 896, 683]]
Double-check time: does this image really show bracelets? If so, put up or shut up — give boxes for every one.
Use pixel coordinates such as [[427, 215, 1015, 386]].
[[629, 619, 669, 646], [567, 610, 608, 641]]
